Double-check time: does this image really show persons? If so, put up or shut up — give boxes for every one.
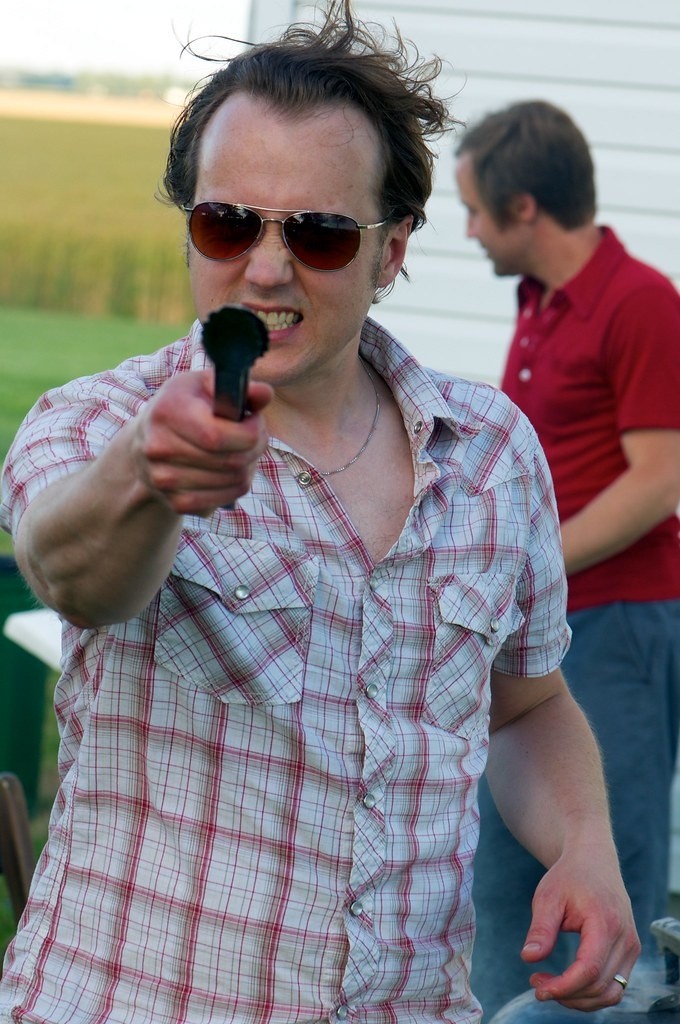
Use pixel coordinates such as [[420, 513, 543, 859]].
[[0, 0, 642, 1024], [452, 101, 679, 1023]]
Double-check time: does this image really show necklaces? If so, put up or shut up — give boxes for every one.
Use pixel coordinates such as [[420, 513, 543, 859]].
[[319, 355, 380, 476]]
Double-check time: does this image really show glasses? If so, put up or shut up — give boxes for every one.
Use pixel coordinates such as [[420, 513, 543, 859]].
[[176, 202, 392, 273]]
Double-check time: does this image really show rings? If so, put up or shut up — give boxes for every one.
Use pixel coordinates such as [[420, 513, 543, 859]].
[[613, 974, 628, 990]]
